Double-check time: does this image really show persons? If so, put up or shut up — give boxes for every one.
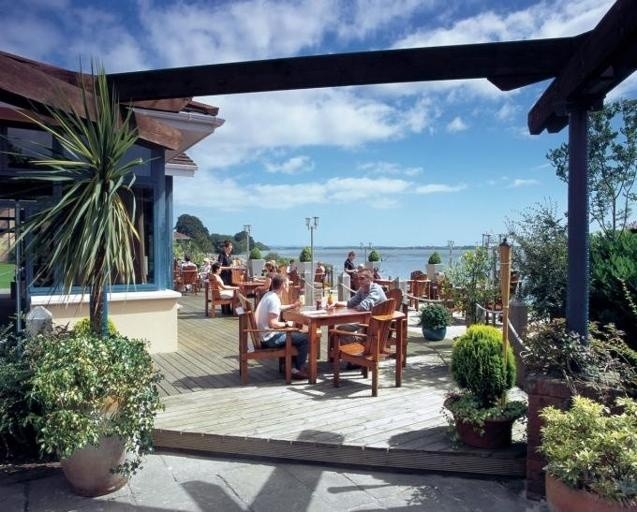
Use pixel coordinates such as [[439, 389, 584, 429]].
[[172, 240, 383, 316], [256, 275, 308, 379], [328, 269, 387, 370]]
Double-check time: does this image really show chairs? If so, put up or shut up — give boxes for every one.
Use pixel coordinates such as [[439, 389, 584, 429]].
[[180, 265, 428, 397]]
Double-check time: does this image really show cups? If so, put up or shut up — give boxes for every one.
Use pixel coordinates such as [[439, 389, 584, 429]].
[[328, 292, 334, 307], [316, 298, 323, 311]]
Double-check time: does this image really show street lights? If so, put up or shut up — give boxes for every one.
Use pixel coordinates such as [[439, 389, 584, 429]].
[[306, 217, 321, 305], [244, 223, 252, 277]]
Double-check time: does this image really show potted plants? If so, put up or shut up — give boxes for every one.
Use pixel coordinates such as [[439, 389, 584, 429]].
[[367, 250, 380, 270], [19, 328, 165, 497], [246, 246, 315, 282], [441, 323, 636, 511], [426, 252, 443, 280], [416, 304, 451, 341]]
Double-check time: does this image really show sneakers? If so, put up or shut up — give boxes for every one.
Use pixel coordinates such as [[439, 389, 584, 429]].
[[291, 367, 310, 379]]
